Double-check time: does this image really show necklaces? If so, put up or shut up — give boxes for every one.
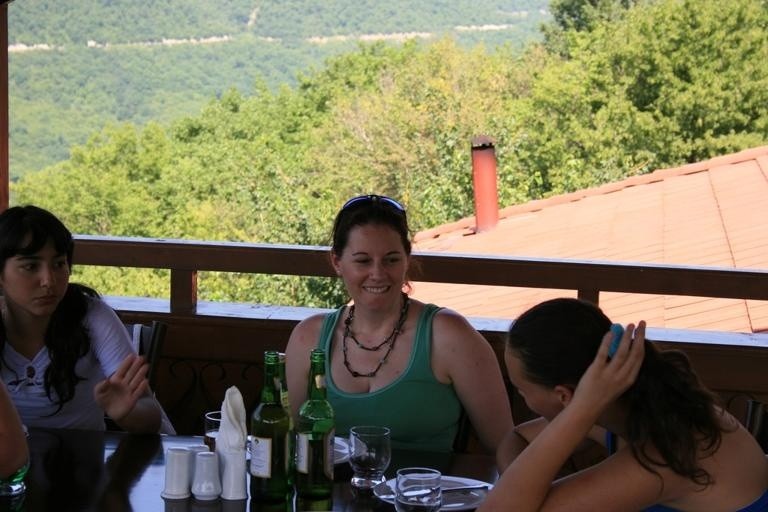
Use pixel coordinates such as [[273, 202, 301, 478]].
[[342, 290, 410, 377]]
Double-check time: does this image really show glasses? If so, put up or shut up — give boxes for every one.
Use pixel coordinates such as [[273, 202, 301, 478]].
[[340, 194, 406, 224]]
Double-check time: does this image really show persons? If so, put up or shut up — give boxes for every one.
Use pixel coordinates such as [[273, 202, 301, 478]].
[[0, 203, 163, 434], [0, 379, 28, 481], [473, 296, 768, 512], [283, 192, 515, 458]]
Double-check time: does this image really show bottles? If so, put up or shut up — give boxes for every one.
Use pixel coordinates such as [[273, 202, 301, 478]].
[[158, 444, 248, 502], [249, 348, 335, 501]]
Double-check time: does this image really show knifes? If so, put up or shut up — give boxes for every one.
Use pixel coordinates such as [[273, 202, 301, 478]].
[[373, 483, 488, 500]]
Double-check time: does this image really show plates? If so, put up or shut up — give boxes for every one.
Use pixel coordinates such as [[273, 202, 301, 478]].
[[336, 436, 368, 465], [372, 474, 495, 511]]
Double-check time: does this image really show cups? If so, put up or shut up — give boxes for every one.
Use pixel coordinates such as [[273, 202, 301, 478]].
[[204, 410, 223, 452], [0, 424, 30, 497], [348, 426, 442, 511]]
